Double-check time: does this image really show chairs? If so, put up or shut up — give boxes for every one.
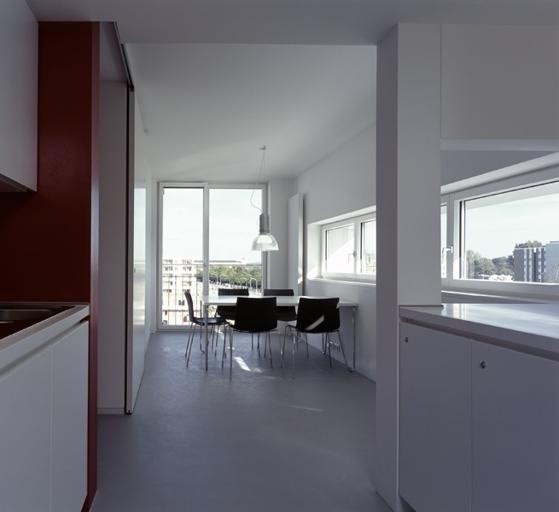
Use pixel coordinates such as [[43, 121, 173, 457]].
[[215, 288, 257, 351], [183, 289, 234, 368], [221, 297, 276, 375], [263, 289, 300, 356], [281, 297, 350, 371]]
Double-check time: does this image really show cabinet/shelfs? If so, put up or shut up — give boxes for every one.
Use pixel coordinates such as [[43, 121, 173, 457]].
[[398, 321, 559, 512], [1, 307, 88, 510]]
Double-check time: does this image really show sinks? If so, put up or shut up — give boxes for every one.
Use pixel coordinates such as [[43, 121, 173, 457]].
[[0, 302, 75, 323], [0, 321, 34, 340]]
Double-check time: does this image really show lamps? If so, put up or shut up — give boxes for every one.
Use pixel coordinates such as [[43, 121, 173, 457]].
[[249, 146, 279, 251]]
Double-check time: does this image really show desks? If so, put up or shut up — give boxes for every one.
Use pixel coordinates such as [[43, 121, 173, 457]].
[[199, 295, 359, 380]]
